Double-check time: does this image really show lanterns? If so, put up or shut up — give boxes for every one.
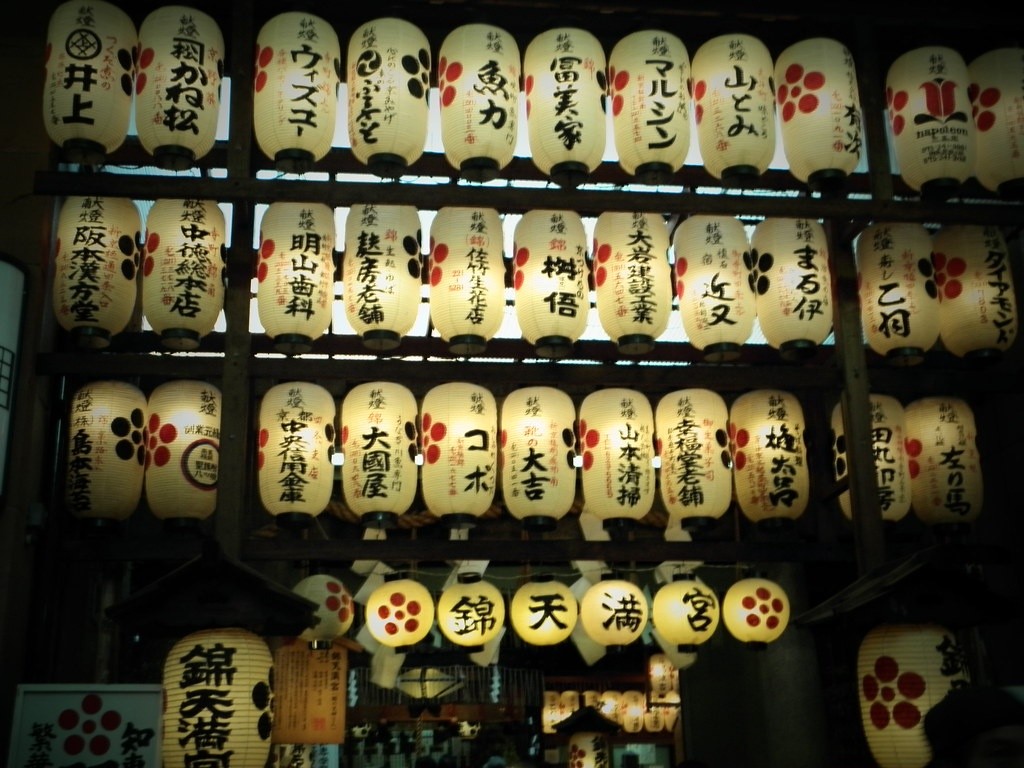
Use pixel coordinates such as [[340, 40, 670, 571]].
[[858, 219, 1019, 368], [884, 39, 1024, 200], [68, 372, 222, 526], [40, 0, 861, 190], [365, 572, 435, 654], [722, 569, 791, 650], [161, 623, 277, 768], [651, 573, 720, 652], [256, 377, 810, 528], [437, 572, 505, 652], [543, 654, 681, 733], [54, 196, 832, 361], [291, 575, 354, 649], [580, 572, 648, 651], [829, 389, 984, 524], [510, 575, 578, 652], [568, 730, 610, 768], [857, 624, 971, 768]]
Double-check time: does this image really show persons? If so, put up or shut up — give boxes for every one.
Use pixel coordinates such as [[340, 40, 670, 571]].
[[925, 687, 1024, 768], [415, 754, 458, 768], [484, 750, 506, 768]]
